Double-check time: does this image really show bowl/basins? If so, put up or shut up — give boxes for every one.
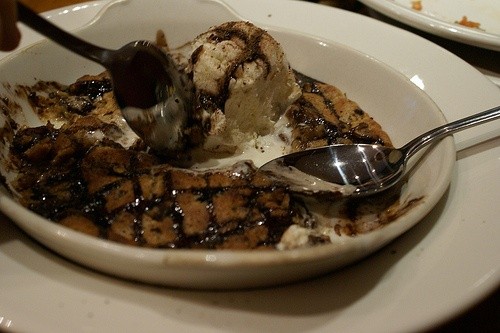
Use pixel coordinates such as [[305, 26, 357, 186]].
[[1, 0, 455, 288]]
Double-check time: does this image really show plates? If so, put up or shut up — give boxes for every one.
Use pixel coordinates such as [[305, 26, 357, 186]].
[[1, 0, 500, 333], [359, 0, 500, 50]]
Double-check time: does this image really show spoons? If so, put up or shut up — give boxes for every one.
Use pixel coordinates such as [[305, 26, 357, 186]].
[[16, 1, 204, 169], [256, 106, 500, 197]]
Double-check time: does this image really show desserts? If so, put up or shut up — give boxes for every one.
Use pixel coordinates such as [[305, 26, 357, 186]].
[[10, 19, 402, 252]]
